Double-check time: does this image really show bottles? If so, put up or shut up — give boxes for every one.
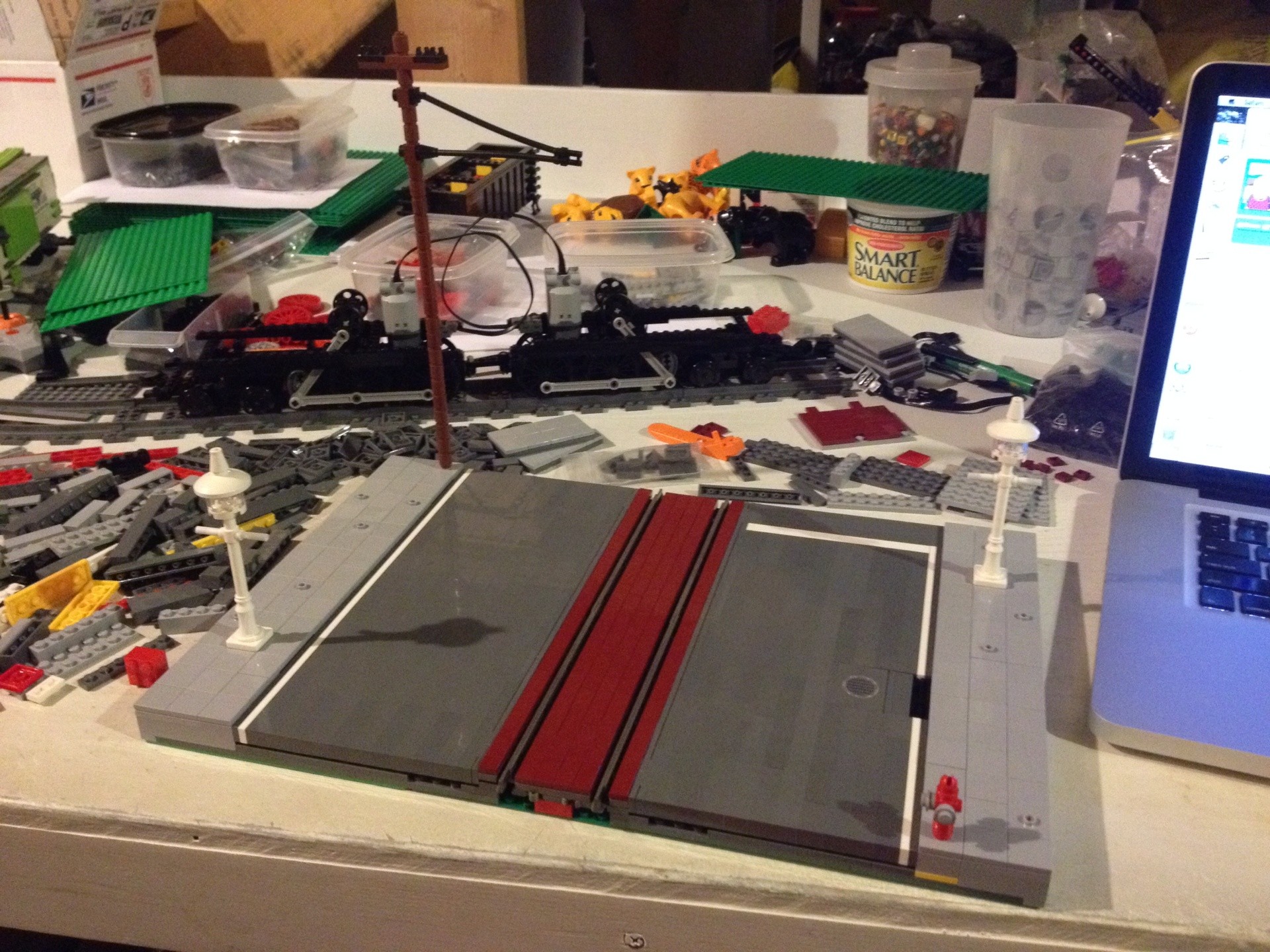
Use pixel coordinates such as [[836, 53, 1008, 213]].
[[863, 43, 983, 172]]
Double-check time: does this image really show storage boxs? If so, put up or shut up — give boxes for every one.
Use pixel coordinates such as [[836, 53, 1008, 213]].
[[0, 0, 165, 186]]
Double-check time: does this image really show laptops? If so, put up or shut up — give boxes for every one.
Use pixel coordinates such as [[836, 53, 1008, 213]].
[[1087, 61, 1270, 781]]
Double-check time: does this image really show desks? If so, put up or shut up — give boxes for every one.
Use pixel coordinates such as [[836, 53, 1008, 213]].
[[0, 78, 1270, 952]]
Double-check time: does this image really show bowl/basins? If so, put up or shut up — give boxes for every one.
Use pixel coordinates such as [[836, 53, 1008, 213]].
[[333, 212, 521, 326], [88, 102, 241, 188], [543, 219, 736, 312], [201, 101, 356, 191]]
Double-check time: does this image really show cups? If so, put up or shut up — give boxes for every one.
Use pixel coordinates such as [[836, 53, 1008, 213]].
[[846, 196, 961, 294], [983, 103, 1133, 338], [1015, 47, 1126, 108]]
